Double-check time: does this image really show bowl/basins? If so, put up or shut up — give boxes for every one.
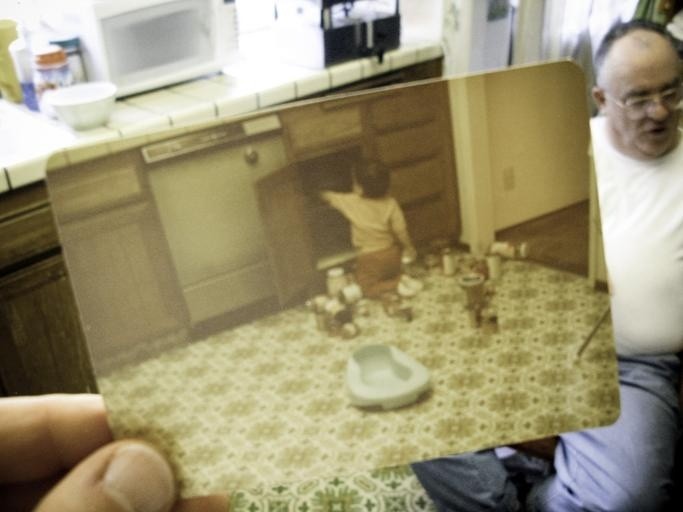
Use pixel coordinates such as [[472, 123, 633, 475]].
[[47, 81, 116, 131]]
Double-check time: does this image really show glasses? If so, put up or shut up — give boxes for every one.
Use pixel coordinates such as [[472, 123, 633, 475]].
[[599, 88, 680, 118]]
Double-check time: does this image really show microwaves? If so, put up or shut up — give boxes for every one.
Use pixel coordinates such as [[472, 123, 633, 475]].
[[71, 0, 244, 99]]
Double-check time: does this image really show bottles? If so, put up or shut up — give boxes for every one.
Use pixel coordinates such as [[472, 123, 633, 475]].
[[9, 35, 87, 113], [306, 239, 528, 335]]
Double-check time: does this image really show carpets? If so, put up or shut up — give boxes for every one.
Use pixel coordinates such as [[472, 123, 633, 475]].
[[99, 256, 619, 499]]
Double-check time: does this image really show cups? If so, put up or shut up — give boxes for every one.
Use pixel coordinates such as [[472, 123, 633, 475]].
[[0, 20, 24, 103]]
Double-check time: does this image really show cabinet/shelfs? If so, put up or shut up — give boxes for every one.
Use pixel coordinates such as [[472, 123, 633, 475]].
[[0, 178, 96, 393], [247, 101, 367, 308], [362, 80, 462, 246], [45, 148, 195, 378]]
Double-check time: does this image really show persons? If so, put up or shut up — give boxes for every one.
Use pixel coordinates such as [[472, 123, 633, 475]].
[[310, 160, 416, 322], [406, 17, 682, 512], [0, 393, 230, 512]]
